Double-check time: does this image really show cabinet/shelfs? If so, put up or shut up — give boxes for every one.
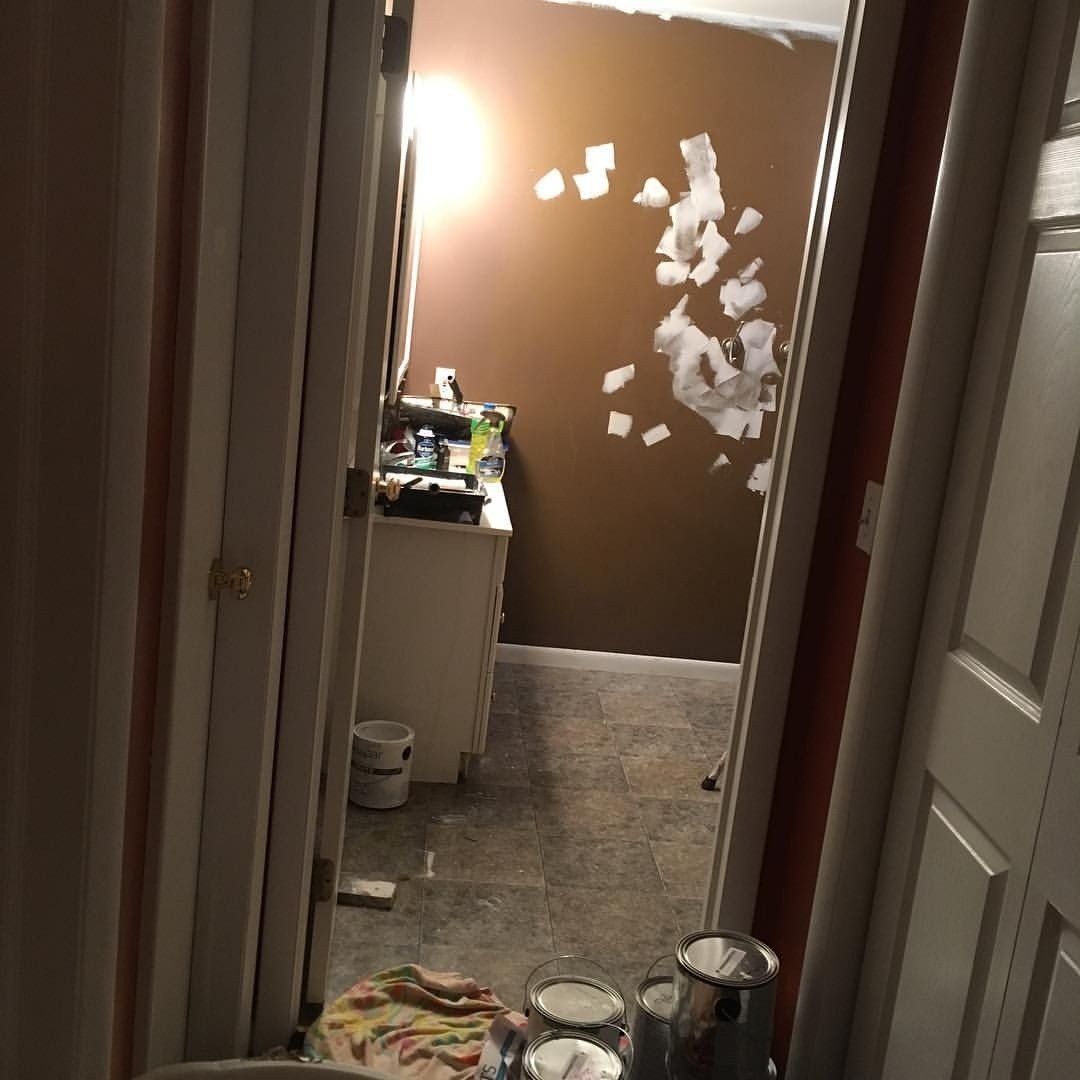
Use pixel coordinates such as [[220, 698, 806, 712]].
[[353, 472, 514, 786]]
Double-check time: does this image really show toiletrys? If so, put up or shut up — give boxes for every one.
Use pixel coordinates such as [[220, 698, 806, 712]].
[[474, 426, 506, 482]]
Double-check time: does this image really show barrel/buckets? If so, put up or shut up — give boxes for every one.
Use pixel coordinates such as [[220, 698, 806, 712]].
[[353, 721, 416, 811], [667, 928, 779, 1079], [521, 956, 629, 1053], [631, 955, 683, 1080], [516, 1029, 627, 1080]]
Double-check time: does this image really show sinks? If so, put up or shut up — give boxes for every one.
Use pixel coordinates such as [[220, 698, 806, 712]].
[[129, 1057, 396, 1080]]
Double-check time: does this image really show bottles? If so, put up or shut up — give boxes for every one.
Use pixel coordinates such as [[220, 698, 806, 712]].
[[468, 417, 489, 473], [475, 411, 506, 483], [437, 439, 450, 471]]
